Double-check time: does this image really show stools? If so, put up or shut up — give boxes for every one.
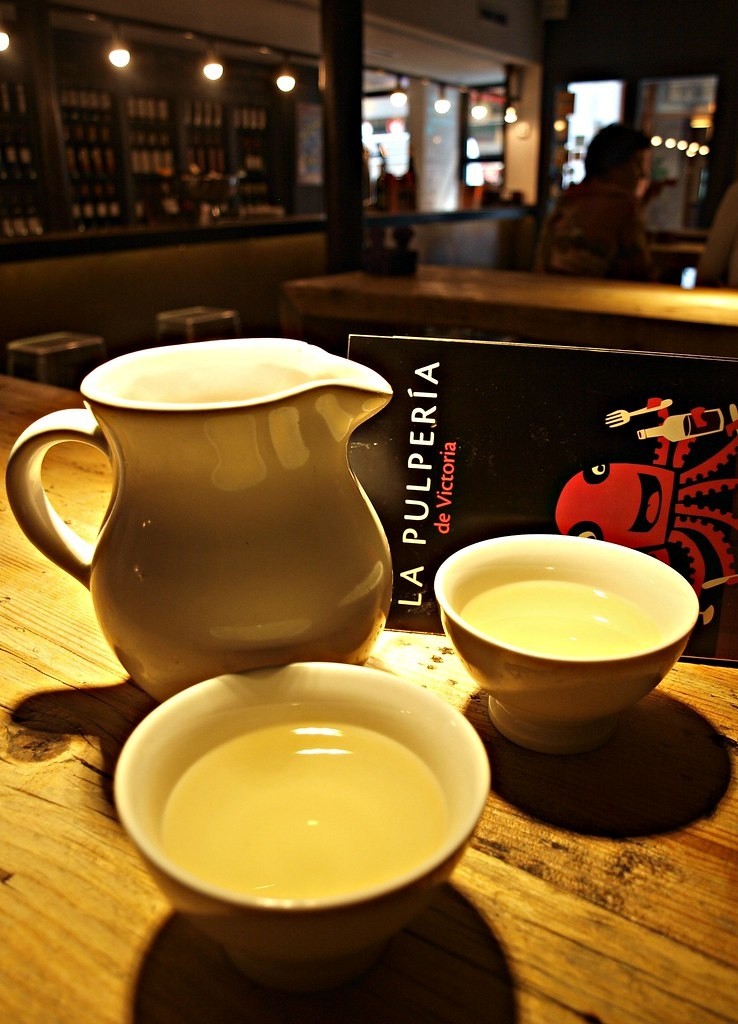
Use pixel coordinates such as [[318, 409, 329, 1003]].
[[7, 331, 107, 384], [155, 306, 243, 346]]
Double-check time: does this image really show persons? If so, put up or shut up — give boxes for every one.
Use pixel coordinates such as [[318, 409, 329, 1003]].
[[699, 177, 738, 287], [539, 123, 662, 282]]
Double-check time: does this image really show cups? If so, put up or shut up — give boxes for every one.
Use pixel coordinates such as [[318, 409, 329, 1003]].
[[111, 660, 494, 986], [432, 530, 700, 756]]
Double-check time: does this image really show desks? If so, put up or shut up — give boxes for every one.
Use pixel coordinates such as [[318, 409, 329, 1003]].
[[647, 239, 704, 268], [0, 375, 738, 1024], [278, 266, 738, 360]]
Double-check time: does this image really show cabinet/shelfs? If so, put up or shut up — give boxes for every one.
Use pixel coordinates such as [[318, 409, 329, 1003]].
[[0, 19, 298, 239]]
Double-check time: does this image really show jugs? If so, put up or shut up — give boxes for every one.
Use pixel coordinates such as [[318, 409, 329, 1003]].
[[4, 336, 396, 708]]
